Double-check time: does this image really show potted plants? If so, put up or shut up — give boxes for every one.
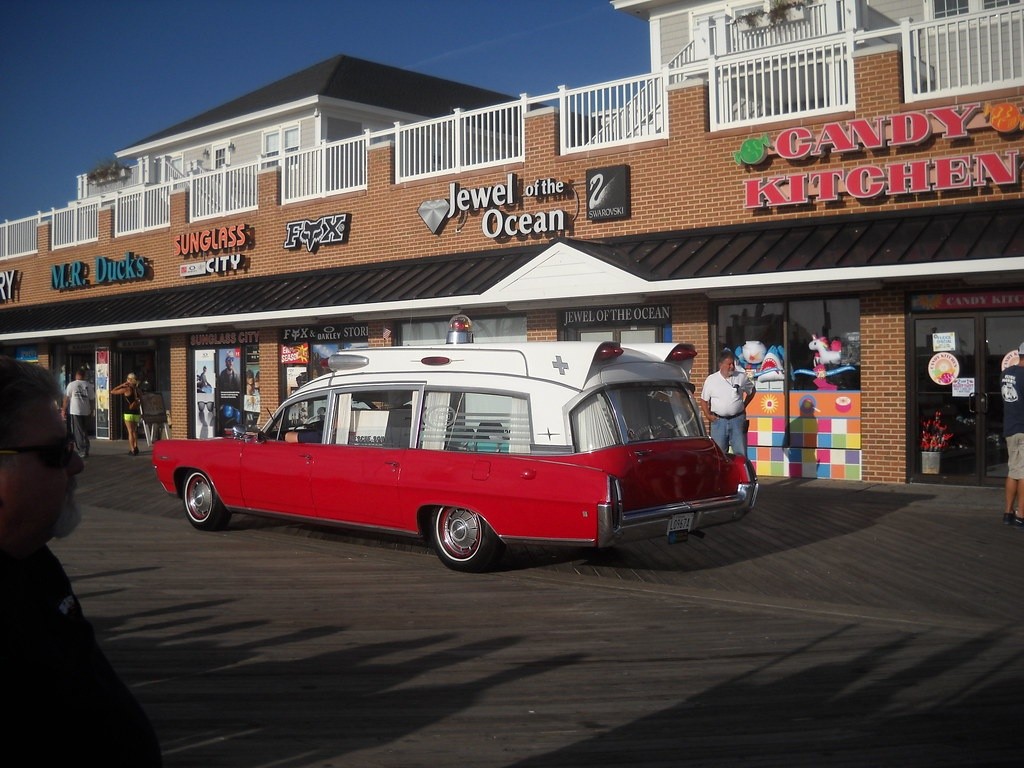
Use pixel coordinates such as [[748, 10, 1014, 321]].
[[730, 0, 809, 32], [87, 157, 131, 185]]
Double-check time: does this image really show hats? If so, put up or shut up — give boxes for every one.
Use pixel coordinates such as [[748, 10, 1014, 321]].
[[1018, 342, 1024, 354]]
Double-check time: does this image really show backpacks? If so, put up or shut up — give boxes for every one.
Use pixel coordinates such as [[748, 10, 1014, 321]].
[[125, 384, 141, 410]]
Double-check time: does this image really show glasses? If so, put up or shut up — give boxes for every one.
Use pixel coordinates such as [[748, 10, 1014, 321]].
[[0, 430, 77, 469]]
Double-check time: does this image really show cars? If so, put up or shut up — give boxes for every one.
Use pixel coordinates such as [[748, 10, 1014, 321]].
[[152, 314, 760, 568]]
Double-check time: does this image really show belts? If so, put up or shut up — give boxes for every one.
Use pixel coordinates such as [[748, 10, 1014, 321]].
[[711, 410, 745, 419]]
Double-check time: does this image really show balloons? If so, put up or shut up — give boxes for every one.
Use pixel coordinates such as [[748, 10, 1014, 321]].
[[235, 410, 242, 425], [223, 402, 235, 419]]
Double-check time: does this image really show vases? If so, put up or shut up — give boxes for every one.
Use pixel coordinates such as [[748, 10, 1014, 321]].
[[919, 452, 940, 474]]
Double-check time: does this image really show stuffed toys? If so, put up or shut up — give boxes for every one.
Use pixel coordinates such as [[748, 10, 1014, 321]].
[[733, 333, 841, 390]]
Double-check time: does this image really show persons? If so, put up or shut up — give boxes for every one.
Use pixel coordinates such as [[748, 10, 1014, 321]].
[[1, 356, 165, 768], [1000, 340, 1024, 531], [197, 366, 212, 390], [219, 349, 240, 391], [246, 369, 259, 394], [111, 372, 143, 456], [60, 367, 96, 460], [285, 418, 325, 445], [700, 349, 757, 457]]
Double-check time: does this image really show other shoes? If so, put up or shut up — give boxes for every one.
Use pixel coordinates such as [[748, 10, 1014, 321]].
[[1014, 517, 1024, 529], [78, 451, 89, 458], [1002, 511, 1015, 525], [129, 447, 139, 455]]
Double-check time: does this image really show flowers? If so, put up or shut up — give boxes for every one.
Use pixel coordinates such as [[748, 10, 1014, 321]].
[[921, 411, 954, 451]]
